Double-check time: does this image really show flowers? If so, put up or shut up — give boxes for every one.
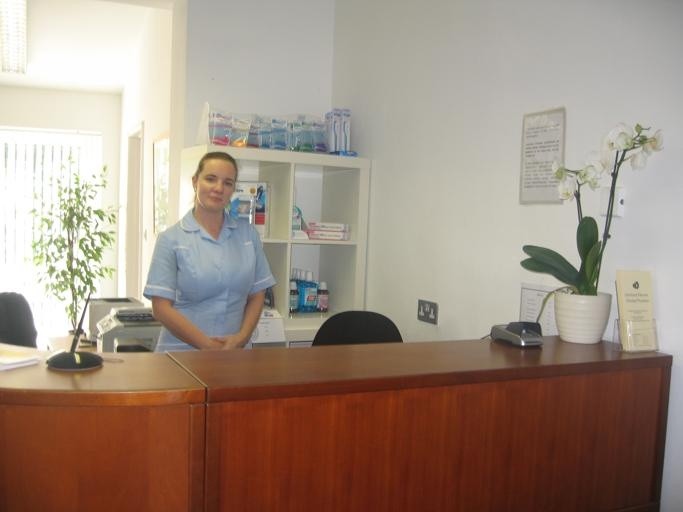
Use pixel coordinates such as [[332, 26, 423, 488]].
[[518, 124, 665, 322]]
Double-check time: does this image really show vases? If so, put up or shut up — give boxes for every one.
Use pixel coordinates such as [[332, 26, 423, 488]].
[[554, 290, 612, 344]]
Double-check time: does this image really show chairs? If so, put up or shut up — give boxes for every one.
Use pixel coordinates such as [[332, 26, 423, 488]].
[[0, 292, 38, 348], [311, 310, 402, 346]]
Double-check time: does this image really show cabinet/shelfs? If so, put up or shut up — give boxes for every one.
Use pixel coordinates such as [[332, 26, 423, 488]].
[[178, 143, 371, 347]]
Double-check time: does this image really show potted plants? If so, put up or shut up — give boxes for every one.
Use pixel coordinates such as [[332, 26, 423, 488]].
[[24, 151, 122, 335]]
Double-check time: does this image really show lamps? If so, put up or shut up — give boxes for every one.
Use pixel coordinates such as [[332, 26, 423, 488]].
[[0, 0, 28, 77]]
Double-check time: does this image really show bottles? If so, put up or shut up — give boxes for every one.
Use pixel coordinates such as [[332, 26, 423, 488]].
[[289, 266, 329, 314]]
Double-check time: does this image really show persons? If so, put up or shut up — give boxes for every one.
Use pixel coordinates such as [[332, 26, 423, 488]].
[[141, 151, 279, 354]]
